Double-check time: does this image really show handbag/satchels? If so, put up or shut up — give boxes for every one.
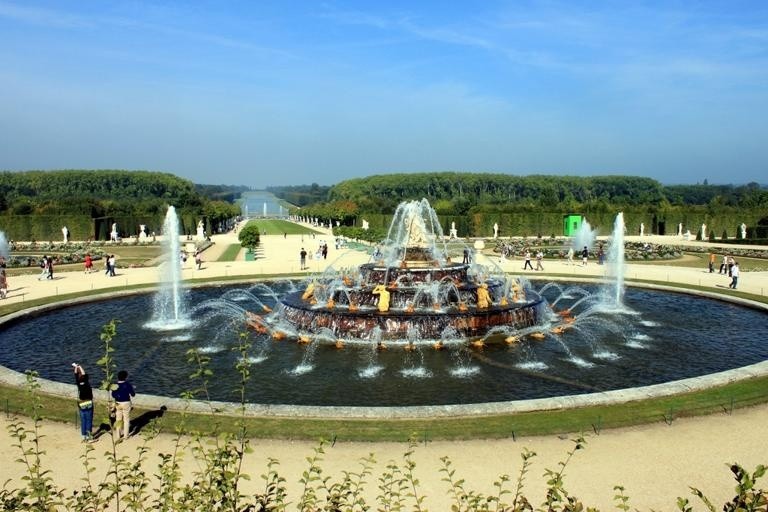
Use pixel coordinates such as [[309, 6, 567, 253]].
[[77, 400, 93, 410]]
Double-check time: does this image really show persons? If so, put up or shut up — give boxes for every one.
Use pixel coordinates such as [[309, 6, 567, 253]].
[[462, 243, 740, 289], [284, 232, 347, 270], [71, 362, 95, 441], [0, 253, 117, 299], [110, 369, 135, 442], [180, 230, 212, 270], [236, 224, 239, 230]]
